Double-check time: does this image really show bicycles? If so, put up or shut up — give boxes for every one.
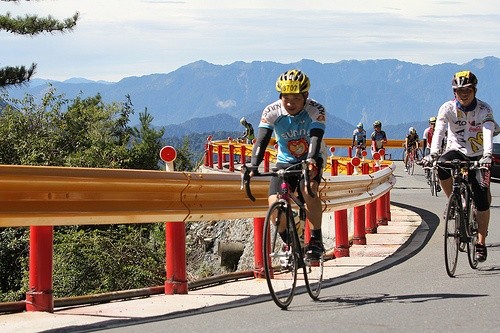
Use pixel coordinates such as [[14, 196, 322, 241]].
[[239, 159, 326, 309], [402, 145, 442, 198], [422, 159, 494, 278]]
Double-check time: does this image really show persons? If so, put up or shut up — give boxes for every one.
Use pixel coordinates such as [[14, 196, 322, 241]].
[[240, 117, 254, 145], [353, 122, 366, 158], [371, 121, 387, 160], [421, 70, 495, 262], [421, 117, 444, 193], [405, 127, 419, 171], [240, 70, 327, 272]]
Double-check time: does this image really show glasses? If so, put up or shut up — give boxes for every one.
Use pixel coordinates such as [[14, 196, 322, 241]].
[[430, 123, 435, 125]]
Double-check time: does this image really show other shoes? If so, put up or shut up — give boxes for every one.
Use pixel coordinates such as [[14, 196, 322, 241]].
[[306, 240, 325, 258], [404, 167, 407, 171], [436, 184, 442, 192]]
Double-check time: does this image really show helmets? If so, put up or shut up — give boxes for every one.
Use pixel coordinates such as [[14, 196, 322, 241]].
[[373, 121, 382, 129], [429, 117, 437, 123], [240, 118, 246, 124], [357, 123, 363, 128], [276, 69, 311, 93], [452, 71, 478, 90], [409, 128, 416, 134]]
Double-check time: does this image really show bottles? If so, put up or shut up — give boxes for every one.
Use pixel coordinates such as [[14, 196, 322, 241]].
[[291, 208, 305, 249], [469, 198, 473, 223]]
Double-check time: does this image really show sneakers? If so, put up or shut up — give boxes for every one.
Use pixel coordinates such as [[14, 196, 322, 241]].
[[475, 243, 487, 262], [443, 203, 455, 219]]
